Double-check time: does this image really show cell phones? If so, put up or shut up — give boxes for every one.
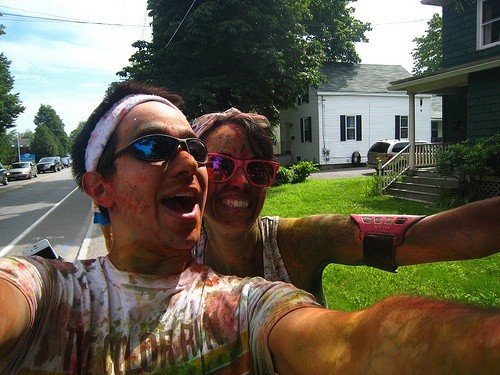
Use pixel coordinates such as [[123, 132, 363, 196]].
[[23, 239, 58, 260]]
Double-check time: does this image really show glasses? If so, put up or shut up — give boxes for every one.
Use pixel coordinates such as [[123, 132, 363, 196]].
[[205, 153, 280, 188], [112, 133, 208, 166]]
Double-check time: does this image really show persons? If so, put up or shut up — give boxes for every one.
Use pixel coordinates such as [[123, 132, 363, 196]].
[[188, 104, 500, 312], [2, 80, 500, 375]]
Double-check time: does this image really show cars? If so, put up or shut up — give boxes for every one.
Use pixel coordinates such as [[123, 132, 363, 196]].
[[365, 137, 438, 173], [61, 155, 73, 168], [0, 162, 8, 185], [6, 161, 38, 182], [37, 156, 62, 175]]
